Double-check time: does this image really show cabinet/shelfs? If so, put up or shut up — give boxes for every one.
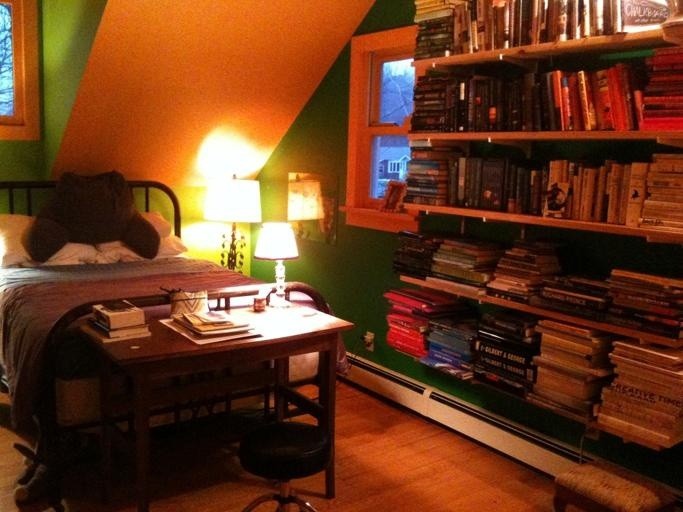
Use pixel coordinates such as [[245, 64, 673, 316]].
[[394, 28, 683, 498]]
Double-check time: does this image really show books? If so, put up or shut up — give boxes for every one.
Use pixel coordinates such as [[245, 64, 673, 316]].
[[412, 0, 679, 61], [411, 62, 677, 132], [79, 296, 150, 344], [399, 228, 679, 339], [405, 138, 677, 228], [161, 310, 263, 347]]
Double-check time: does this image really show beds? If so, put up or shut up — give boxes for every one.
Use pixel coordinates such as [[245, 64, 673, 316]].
[[1, 177, 335, 500]]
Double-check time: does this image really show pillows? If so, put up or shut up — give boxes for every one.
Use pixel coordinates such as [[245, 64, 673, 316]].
[[90, 240, 190, 266], [27, 169, 160, 260], [95, 208, 171, 252], [0, 212, 97, 267]]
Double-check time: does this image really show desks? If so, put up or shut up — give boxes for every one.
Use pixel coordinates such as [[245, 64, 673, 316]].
[[78, 298, 355, 512]]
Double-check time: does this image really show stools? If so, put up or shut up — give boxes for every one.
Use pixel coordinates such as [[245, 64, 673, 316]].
[[237, 419, 330, 512]]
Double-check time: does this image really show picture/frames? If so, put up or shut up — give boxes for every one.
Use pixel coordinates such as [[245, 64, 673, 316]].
[[0, 0, 46, 143], [382, 181, 406, 213]]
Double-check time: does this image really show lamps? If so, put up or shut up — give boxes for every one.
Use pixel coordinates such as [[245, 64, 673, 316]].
[[286, 178, 334, 242], [203, 178, 261, 272], [253, 222, 299, 298]]
